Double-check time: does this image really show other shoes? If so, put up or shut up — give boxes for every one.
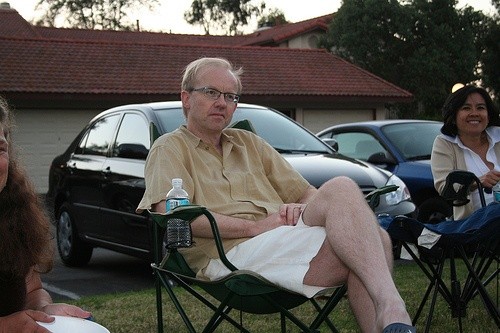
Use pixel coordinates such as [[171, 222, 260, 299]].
[[383, 322, 415, 333]]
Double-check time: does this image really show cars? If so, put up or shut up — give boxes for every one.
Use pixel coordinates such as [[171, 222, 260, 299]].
[[48, 101, 417, 280], [297, 119, 455, 241]]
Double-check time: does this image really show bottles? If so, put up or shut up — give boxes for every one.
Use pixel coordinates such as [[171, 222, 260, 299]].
[[166, 178, 192, 247], [491, 180, 500, 204]]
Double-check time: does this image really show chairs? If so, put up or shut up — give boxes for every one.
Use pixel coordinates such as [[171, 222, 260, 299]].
[[405, 140, 428, 154], [356, 140, 380, 153], [147, 171, 500, 333]]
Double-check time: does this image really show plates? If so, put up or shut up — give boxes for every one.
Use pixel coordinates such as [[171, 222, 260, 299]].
[[35, 314, 110, 333]]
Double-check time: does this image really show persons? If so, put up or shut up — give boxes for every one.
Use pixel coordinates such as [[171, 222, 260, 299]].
[[135, 58, 415, 333], [0, 95, 91, 333], [432, 84, 500, 221]]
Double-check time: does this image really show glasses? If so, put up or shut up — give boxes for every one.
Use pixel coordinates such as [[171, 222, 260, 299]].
[[186, 87, 241, 104]]
